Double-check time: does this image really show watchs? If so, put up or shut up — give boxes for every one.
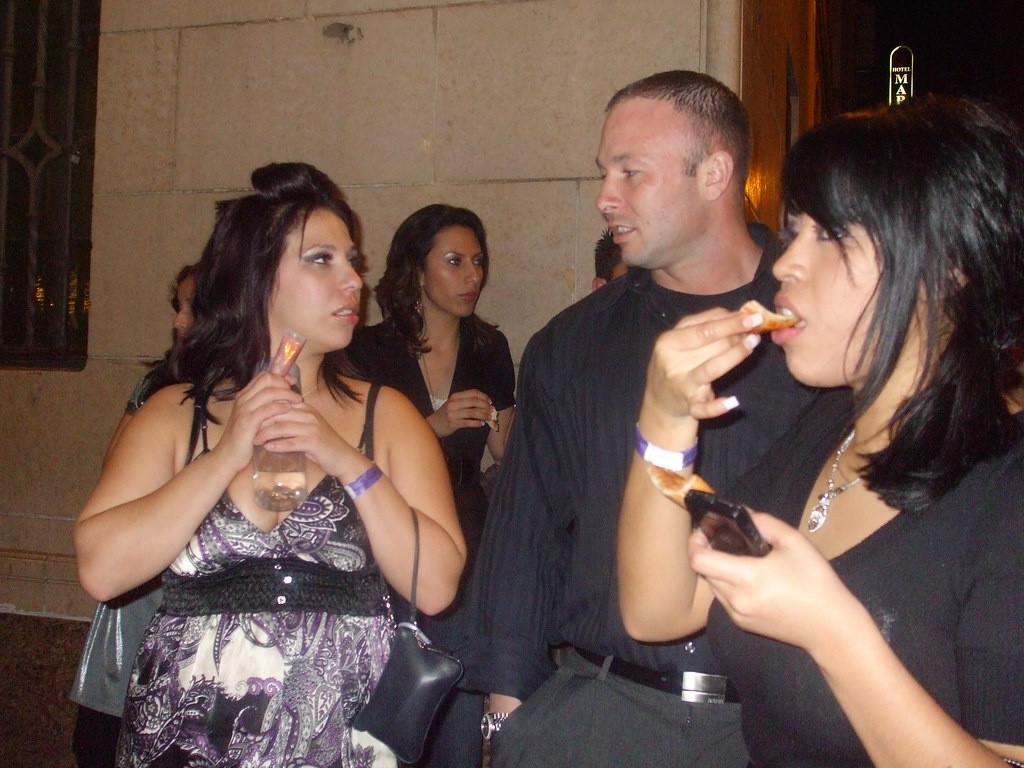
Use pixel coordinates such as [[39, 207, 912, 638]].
[[480, 712, 507, 741]]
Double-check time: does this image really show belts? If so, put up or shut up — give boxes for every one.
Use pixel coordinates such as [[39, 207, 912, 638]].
[[575, 645, 742, 705]]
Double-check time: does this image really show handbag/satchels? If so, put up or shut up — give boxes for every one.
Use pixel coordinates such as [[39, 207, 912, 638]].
[[355, 507, 464, 763]]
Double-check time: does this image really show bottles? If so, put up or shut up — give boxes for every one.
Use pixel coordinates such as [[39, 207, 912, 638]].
[[249, 359, 305, 512]]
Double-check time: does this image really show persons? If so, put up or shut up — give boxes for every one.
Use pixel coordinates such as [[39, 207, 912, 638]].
[[457, 70, 852, 767], [71, 163, 468, 768], [591, 225, 627, 289], [71, 264, 200, 768], [351, 204, 516, 767], [615, 92, 1023, 768]]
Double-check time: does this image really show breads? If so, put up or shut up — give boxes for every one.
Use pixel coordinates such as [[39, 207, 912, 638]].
[[739, 300, 800, 333], [645, 463, 716, 510]]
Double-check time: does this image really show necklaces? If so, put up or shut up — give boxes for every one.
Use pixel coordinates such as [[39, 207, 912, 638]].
[[806, 429, 863, 533], [421, 352, 436, 411]]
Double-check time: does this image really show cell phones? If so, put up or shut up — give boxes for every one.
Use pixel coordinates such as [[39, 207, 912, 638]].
[[682, 488, 770, 561]]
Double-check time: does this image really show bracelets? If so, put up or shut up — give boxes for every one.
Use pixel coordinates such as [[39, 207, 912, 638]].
[[344, 461, 382, 498], [633, 422, 699, 471]]
